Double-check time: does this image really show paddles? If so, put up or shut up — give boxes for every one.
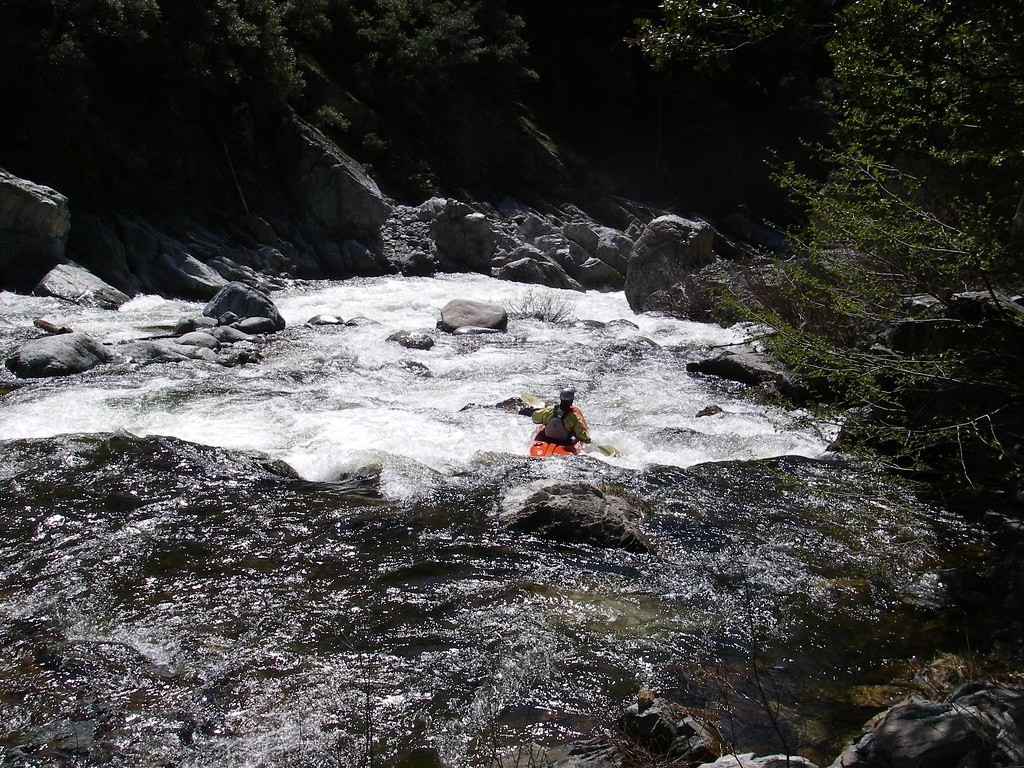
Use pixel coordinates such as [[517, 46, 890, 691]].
[[521, 391, 620, 457]]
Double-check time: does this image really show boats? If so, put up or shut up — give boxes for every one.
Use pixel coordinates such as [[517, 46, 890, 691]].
[[528, 405, 588, 458]]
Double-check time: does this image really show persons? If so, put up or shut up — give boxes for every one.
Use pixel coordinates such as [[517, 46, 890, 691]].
[[532, 387, 591, 446]]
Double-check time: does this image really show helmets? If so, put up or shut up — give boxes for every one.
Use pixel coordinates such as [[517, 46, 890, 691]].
[[560, 389, 574, 401]]
